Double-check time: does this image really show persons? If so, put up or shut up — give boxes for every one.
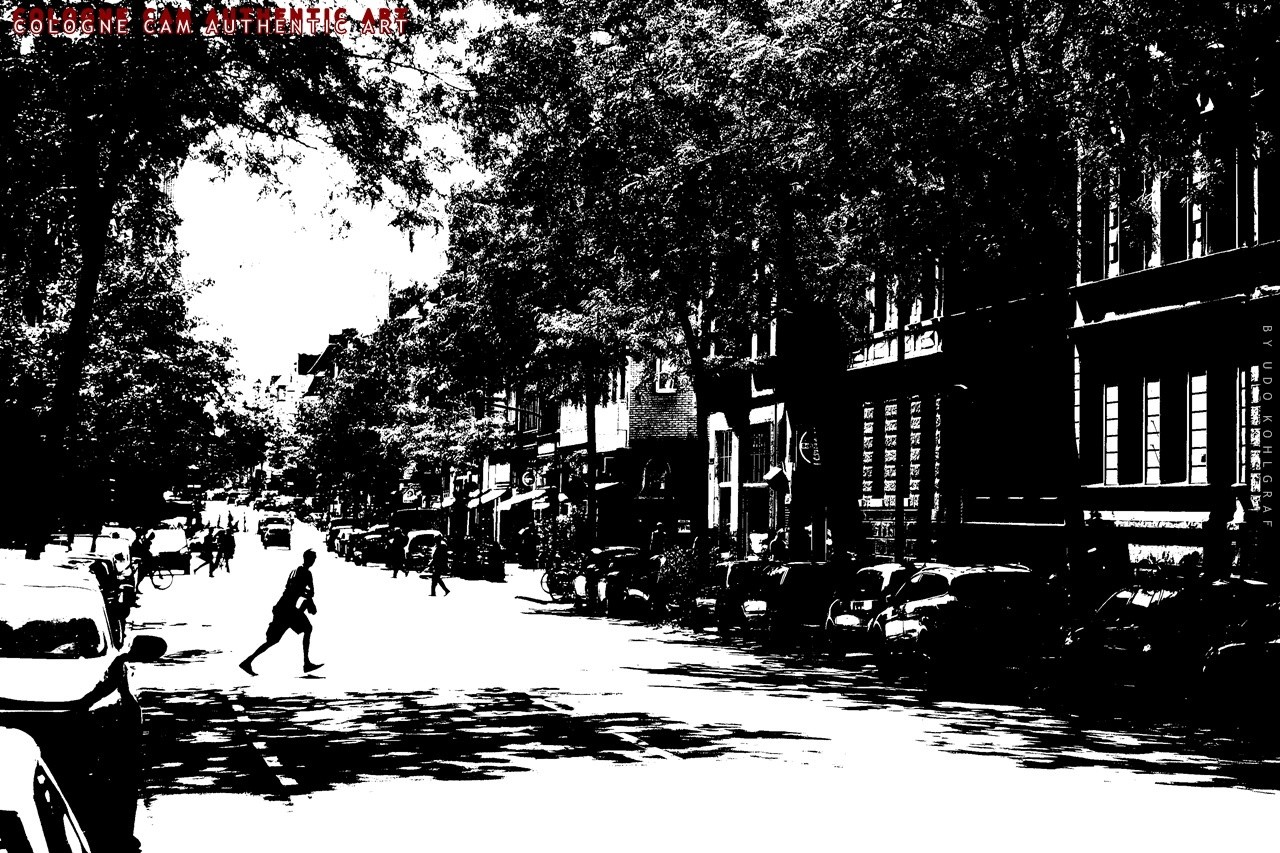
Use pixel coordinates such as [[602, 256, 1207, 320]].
[[430, 535, 450, 596], [131, 530, 158, 594], [390, 527, 409, 579], [193, 526, 235, 577], [239, 549, 323, 676]]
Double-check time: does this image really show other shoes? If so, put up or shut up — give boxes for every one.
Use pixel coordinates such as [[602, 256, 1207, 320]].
[[445, 590, 450, 597], [304, 663, 324, 672], [429, 594, 436, 596], [239, 661, 258, 676]]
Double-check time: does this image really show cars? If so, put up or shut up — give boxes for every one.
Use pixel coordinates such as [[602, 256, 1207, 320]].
[[211, 485, 503, 580], [0, 525, 192, 853], [558, 537, 1280, 743]]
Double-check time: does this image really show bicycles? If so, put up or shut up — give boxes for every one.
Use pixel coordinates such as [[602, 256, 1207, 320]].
[[133, 556, 174, 590]]
